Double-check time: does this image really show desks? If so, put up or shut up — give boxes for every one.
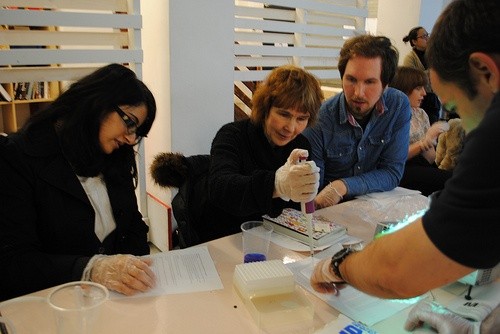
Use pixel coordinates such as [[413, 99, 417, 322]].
[[0, 186, 431, 334]]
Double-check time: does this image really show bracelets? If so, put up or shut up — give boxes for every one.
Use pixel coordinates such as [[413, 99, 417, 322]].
[[473, 322, 481, 334]]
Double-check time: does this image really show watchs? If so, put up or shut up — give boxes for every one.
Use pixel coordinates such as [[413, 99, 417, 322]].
[[330, 248, 362, 281]]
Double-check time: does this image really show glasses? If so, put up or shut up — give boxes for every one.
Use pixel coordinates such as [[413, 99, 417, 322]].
[[113, 105, 144, 139], [416, 33, 429, 39]]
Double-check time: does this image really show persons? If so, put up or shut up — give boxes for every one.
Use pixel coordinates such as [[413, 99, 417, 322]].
[[311, 0, 500, 300], [0, 63, 157, 302], [186, 65, 324, 248], [388, 27, 460, 197], [404, 302, 500, 334], [300, 35, 412, 213]]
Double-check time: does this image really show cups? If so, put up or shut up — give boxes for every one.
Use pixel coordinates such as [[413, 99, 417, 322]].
[[240, 220, 274, 263], [48, 279, 110, 334]]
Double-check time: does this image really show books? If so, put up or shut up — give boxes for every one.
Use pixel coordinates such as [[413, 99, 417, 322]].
[[0, 82, 50, 102], [374, 221, 500, 287], [262, 207, 347, 247]]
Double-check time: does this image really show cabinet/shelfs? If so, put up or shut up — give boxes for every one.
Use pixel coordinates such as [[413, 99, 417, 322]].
[[0, 6, 61, 139]]
